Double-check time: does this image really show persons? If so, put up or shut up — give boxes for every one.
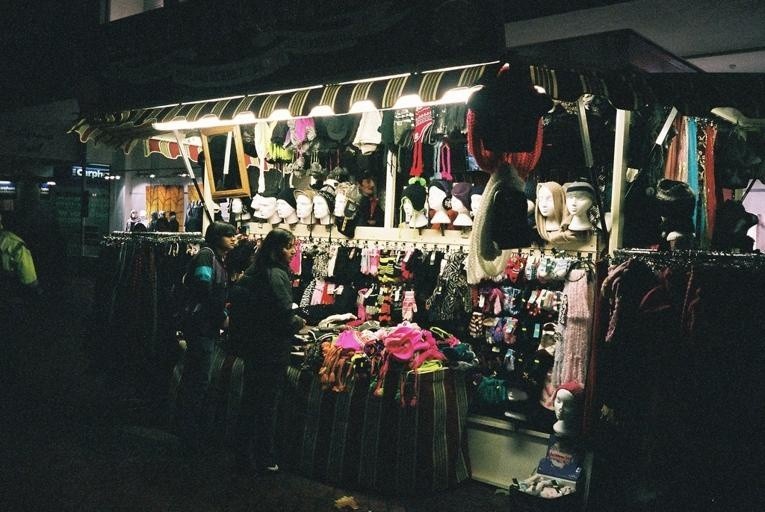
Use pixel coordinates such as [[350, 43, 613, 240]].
[[1, 205, 43, 424], [229, 182, 360, 244], [403, 178, 477, 228], [565, 181, 600, 232], [177, 220, 239, 453], [549, 377, 588, 437], [357, 174, 385, 228], [228, 228, 304, 472], [533, 180, 569, 245], [125, 209, 179, 232]]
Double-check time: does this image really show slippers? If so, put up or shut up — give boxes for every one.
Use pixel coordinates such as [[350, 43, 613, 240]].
[[226, 270, 273, 359]]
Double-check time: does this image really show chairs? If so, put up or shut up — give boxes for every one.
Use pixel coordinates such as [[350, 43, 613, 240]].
[[255, 458, 279, 473]]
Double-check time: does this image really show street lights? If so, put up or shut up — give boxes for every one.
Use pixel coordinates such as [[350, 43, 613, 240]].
[[163, 247, 216, 325]]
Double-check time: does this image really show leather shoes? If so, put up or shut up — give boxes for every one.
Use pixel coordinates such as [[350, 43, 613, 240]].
[[460, 253, 569, 373], [459, 375, 507, 403], [290, 238, 426, 329]]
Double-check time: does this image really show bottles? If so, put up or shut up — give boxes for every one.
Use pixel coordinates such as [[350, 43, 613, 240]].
[[564, 182, 598, 208], [248, 102, 473, 178], [654, 180, 696, 218], [400, 181, 426, 212], [301, 317, 448, 409], [451, 182, 472, 211], [552, 382, 584, 417], [427, 179, 452, 197], [467, 164, 532, 276], [502, 376, 533, 394], [215, 179, 370, 238]]
